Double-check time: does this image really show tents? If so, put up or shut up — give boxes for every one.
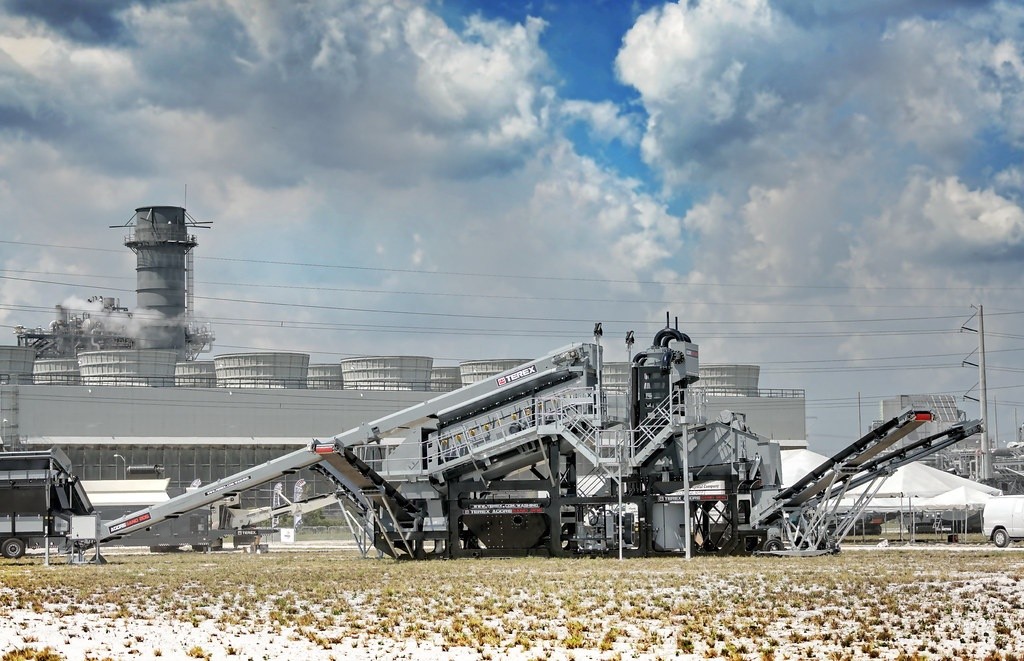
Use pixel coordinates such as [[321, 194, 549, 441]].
[[649, 438, 1002, 541]]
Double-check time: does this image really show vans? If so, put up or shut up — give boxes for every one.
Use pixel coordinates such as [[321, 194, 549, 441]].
[[982, 495, 1023, 548]]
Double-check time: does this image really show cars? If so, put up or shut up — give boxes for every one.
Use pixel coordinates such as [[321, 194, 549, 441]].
[[826, 514, 883, 536]]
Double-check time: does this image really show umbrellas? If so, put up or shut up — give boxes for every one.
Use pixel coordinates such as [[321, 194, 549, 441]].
[[912, 483, 1001, 545]]
